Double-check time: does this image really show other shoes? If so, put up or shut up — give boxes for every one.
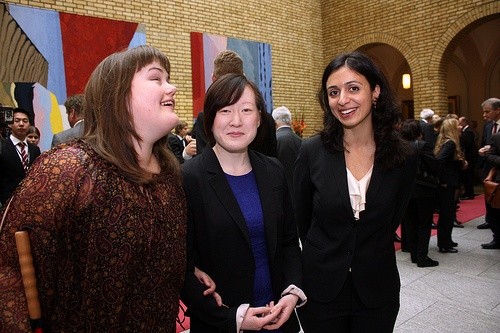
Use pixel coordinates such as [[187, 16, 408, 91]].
[[410, 254, 439, 267]]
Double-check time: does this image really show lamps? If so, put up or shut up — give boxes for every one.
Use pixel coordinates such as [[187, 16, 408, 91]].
[[402, 73, 411, 89]]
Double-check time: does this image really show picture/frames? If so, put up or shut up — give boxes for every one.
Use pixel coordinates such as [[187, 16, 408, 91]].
[[402, 100, 414, 120], [447, 95, 461, 116]]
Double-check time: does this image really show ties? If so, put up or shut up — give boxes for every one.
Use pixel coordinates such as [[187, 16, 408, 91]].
[[18, 142, 29, 171], [493, 122, 497, 133]]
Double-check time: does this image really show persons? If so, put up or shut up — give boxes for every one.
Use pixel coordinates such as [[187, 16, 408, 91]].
[[0, 45, 223, 333], [179, 72, 308, 333], [292, 53, 415, 333], [271, 106, 303, 172], [477, 98, 500, 250], [0, 108, 41, 206], [52, 93, 86, 149], [393, 109, 472, 268], [163, 51, 277, 163]]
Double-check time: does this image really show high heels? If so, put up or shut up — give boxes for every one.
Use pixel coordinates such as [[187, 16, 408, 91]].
[[449, 240, 458, 247], [439, 246, 458, 253]]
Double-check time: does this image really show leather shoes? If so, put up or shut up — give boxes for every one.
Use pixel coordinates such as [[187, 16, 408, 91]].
[[432, 223, 437, 229], [481, 239, 500, 249], [477, 223, 490, 229], [394, 233, 401, 243], [453, 221, 464, 228]]
